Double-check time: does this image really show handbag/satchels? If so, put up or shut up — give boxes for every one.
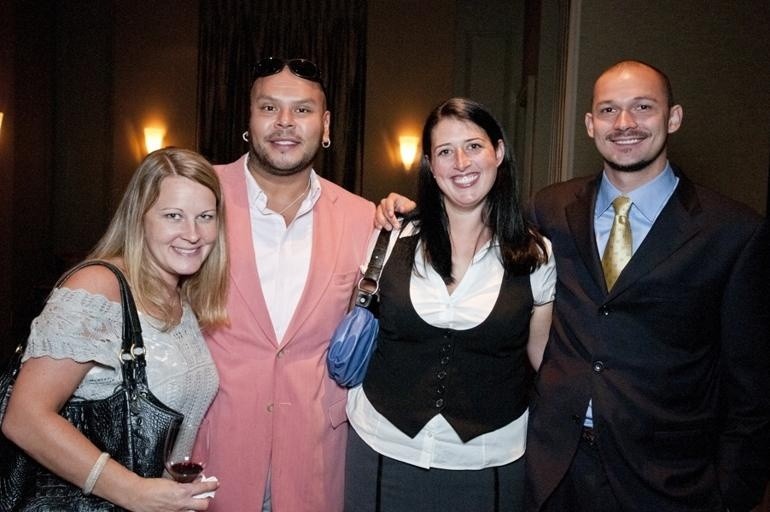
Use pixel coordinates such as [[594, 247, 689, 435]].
[[0, 373, 184, 512], [326, 305, 380, 387]]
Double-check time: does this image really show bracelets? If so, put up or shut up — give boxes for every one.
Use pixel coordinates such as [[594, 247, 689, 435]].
[[83, 451, 111, 495]]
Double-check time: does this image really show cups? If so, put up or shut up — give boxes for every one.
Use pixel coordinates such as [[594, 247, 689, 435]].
[[163, 416, 212, 482]]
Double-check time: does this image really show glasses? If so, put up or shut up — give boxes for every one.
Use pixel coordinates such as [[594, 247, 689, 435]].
[[254, 56, 323, 81]]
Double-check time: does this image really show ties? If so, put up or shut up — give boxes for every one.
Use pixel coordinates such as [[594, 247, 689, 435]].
[[600, 197, 633, 292]]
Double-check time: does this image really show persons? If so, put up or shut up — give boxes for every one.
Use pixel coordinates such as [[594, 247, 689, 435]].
[[0, 145, 232, 512], [373, 60, 770, 512], [188, 55, 378, 512], [344, 98, 558, 511]]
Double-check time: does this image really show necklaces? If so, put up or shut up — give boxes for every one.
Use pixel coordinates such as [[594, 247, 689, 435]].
[[279, 179, 311, 215]]
